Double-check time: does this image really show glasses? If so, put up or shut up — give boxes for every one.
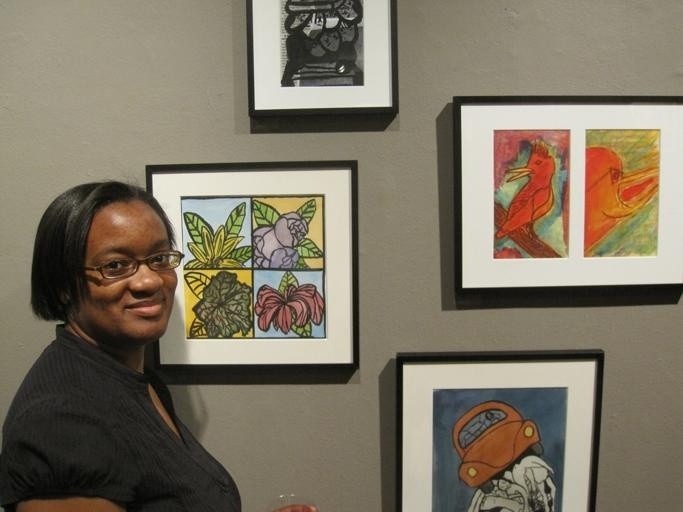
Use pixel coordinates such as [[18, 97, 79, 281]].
[[85, 250, 185, 278]]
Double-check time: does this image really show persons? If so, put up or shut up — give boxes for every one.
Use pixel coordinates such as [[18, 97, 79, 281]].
[[0, 181, 318, 512]]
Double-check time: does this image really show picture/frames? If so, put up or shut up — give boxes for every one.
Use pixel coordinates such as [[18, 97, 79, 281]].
[[452, 95, 683, 297], [393, 348, 605, 512], [145, 159, 359, 371], [246, 0, 399, 117]]
